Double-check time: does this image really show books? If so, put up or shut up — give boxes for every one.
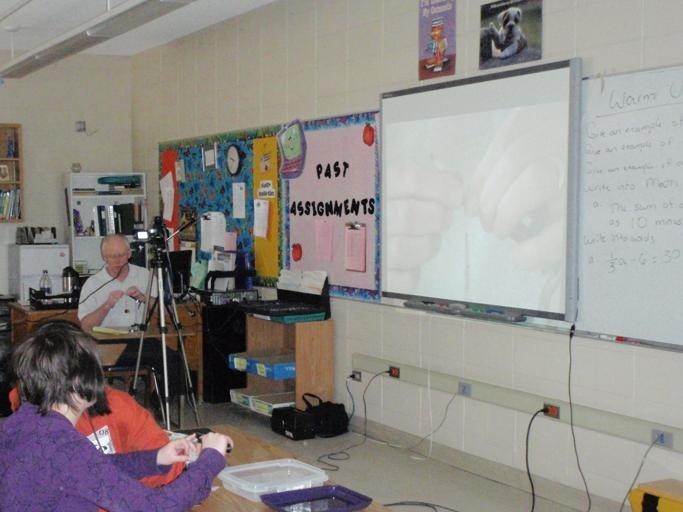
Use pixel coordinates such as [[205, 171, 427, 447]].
[[97, 191, 122, 195], [0, 183, 24, 221], [207, 249, 237, 292], [112, 186, 124, 189], [72, 187, 96, 196]]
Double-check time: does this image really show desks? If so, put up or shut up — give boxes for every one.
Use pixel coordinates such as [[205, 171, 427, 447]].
[[9, 302, 204, 406], [164, 426, 397, 512], [87, 323, 196, 428]]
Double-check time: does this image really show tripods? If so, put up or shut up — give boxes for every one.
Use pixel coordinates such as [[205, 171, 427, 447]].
[[128, 250, 202, 430]]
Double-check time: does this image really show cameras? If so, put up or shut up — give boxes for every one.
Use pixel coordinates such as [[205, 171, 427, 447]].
[[135, 217, 169, 249]]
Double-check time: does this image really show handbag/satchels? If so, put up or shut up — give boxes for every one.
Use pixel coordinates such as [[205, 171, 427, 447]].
[[301, 393, 348, 439]]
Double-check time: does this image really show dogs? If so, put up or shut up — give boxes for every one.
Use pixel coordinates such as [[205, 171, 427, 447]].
[[480, 7, 528, 64]]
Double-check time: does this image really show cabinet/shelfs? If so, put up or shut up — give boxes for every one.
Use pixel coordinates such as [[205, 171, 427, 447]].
[[68, 172, 149, 273], [228, 312, 334, 416], [0, 123, 25, 224]]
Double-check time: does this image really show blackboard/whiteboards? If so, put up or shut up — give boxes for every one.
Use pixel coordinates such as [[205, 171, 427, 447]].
[[377, 62, 683, 353], [378, 56, 582, 323]]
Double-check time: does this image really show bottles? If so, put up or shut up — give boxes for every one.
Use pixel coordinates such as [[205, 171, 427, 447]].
[[62, 266, 79, 302], [40, 270, 52, 304]]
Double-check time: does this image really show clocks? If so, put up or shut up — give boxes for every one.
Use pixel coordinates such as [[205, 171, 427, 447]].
[[226, 144, 242, 176]]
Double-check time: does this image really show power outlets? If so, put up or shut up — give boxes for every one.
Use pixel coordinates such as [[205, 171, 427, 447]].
[[389, 366, 400, 378], [544, 403, 560, 419], [352, 370, 361, 382], [651, 429, 673, 447], [458, 383, 471, 397]]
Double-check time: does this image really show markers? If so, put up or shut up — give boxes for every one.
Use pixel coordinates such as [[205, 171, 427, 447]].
[[598, 334, 625, 342]]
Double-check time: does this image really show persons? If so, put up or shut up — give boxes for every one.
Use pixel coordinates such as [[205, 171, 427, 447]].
[[78, 233, 185, 428], [1, 321, 232, 511], [10, 322, 186, 487]]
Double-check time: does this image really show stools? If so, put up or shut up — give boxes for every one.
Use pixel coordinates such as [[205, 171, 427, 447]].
[[101, 365, 156, 411]]
